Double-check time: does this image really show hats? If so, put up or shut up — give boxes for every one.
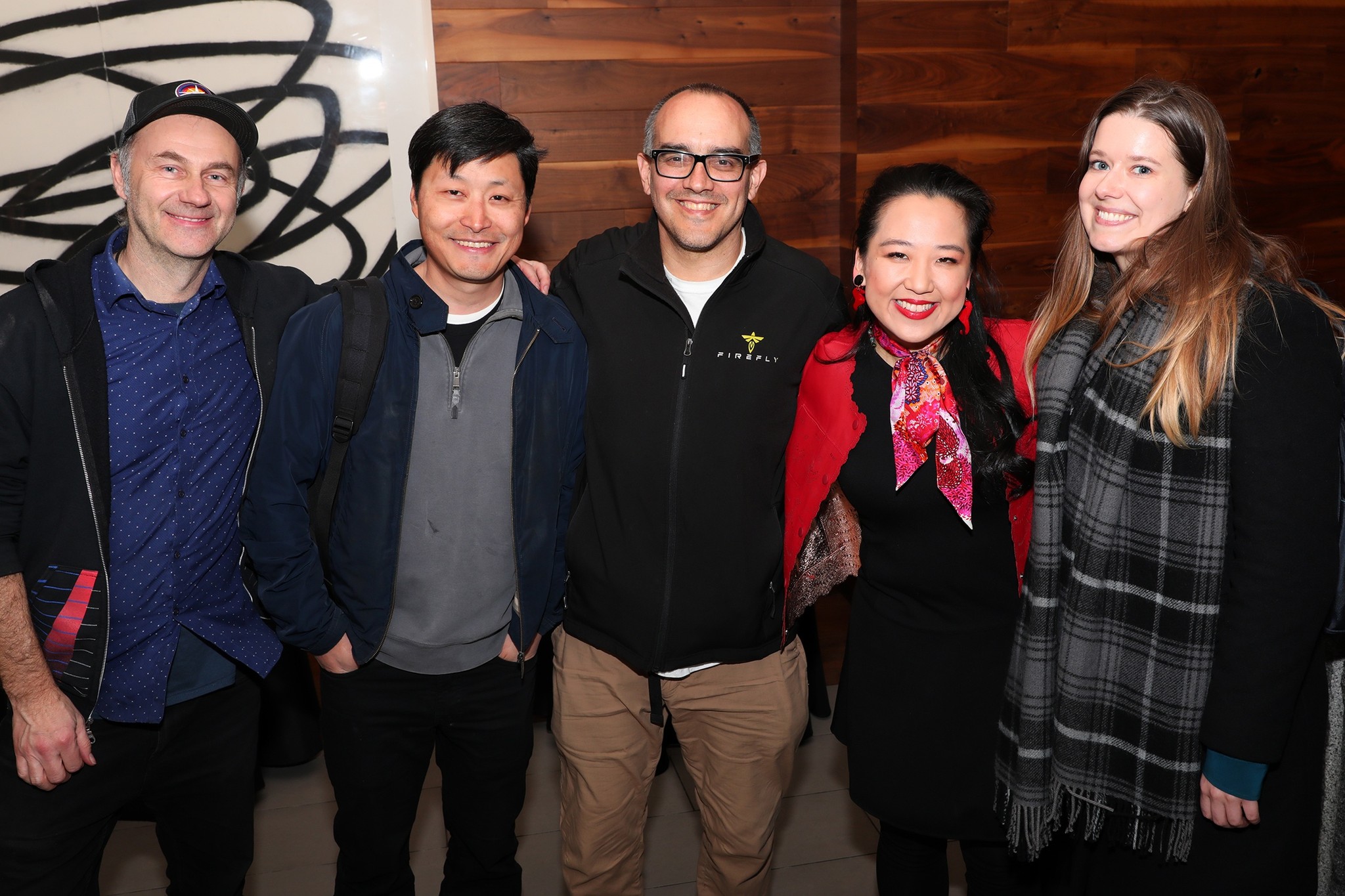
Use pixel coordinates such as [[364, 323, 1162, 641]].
[[119, 80, 259, 164]]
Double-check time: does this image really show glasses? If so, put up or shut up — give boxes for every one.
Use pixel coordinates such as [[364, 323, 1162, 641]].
[[644, 148, 760, 182]]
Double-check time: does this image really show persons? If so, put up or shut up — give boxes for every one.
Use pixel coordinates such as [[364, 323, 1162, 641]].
[[0, 78, 551, 896], [243, 101, 592, 896], [783, 160, 1042, 896], [550, 81, 849, 896], [996, 77, 1345, 896]]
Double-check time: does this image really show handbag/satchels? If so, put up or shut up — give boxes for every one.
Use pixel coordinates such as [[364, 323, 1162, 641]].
[[257, 641, 325, 768]]
